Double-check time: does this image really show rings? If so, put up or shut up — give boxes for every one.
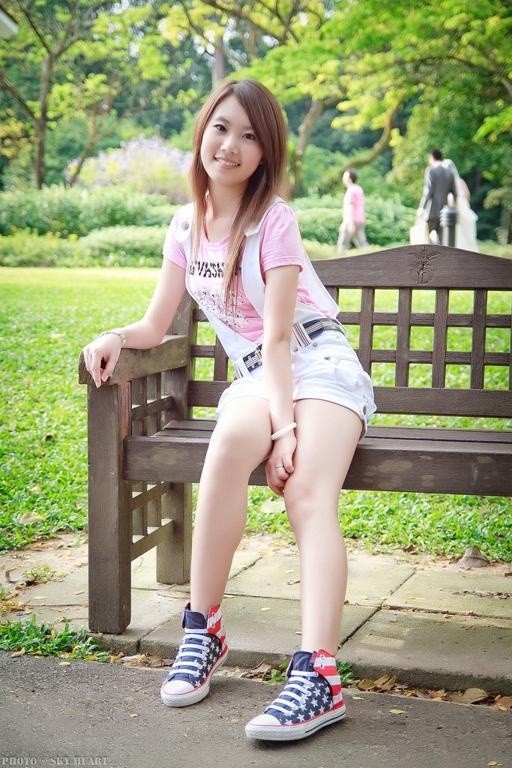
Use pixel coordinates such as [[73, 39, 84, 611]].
[[274, 465, 284, 468]]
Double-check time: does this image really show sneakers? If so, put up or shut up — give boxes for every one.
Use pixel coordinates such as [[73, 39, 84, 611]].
[[160, 601, 229, 708], [245, 648, 347, 742]]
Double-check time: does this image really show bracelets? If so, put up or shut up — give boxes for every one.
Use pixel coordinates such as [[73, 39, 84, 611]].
[[97, 330, 128, 347], [271, 421, 297, 443]]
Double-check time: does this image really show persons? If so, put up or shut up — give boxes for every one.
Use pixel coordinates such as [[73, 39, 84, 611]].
[[82, 76, 378, 744], [335, 168, 371, 255], [440, 159, 472, 252], [416, 148, 458, 245]]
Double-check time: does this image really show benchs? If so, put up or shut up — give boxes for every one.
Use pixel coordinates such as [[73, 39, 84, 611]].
[[78, 243, 512, 635]]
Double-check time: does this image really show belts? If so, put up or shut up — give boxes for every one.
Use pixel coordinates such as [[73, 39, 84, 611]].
[[233, 317, 346, 381]]
